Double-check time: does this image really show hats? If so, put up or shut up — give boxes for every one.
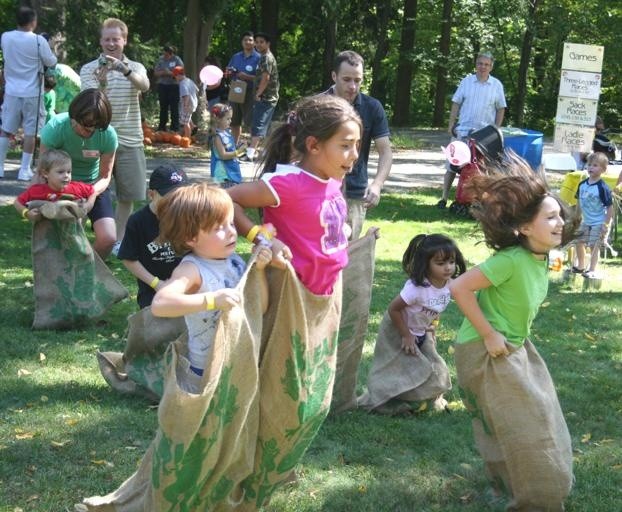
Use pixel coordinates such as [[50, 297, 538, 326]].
[[149, 164, 191, 196], [172, 65, 183, 76]]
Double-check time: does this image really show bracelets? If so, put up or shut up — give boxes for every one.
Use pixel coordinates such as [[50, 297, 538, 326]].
[[431, 320, 439, 327], [604, 222, 609, 228]]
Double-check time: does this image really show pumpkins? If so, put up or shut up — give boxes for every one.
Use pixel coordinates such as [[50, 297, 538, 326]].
[[142, 122, 190, 149]]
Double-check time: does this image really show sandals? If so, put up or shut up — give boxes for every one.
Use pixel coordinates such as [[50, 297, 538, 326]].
[[567, 267, 594, 278]]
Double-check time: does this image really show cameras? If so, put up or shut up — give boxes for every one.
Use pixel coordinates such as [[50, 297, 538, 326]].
[[97, 52, 108, 67]]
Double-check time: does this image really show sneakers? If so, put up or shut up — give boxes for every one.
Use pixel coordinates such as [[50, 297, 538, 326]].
[[438, 200, 447, 209], [17, 168, 35, 182]]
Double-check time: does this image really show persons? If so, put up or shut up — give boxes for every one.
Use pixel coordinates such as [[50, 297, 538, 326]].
[[448, 150, 583, 359], [437, 49, 507, 207], [614, 171, 622, 192], [593, 116, 615, 161], [387, 233, 465, 355], [572, 152, 614, 279]]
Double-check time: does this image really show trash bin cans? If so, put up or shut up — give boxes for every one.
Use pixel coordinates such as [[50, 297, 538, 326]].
[[500, 126, 544, 171]]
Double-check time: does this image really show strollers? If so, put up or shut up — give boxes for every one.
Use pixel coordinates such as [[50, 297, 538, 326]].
[[451, 121, 504, 219]]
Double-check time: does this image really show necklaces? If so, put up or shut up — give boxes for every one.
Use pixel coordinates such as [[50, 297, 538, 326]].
[[521, 246, 547, 255]]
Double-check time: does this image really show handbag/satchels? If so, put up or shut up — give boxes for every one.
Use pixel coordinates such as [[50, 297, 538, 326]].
[[228, 80, 247, 104]]
